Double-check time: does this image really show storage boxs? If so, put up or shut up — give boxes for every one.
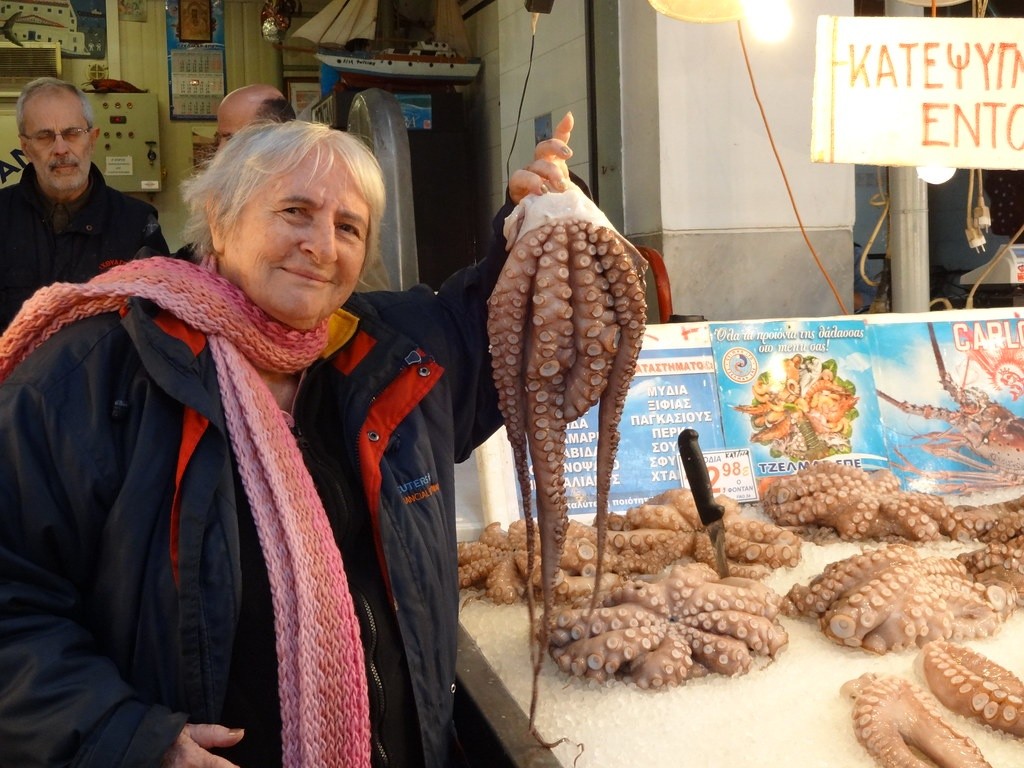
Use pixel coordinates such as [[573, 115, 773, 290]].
[[396, 93, 433, 130]]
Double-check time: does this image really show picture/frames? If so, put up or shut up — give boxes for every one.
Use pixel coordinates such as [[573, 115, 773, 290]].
[[284, 77, 322, 117]]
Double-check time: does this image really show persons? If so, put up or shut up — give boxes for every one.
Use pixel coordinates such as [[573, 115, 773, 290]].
[[0, 76, 170, 335], [175, 83, 296, 267], [0, 111, 585, 768]]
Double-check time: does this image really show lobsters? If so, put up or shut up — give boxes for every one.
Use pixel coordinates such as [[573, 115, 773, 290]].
[[875, 322, 1024, 496]]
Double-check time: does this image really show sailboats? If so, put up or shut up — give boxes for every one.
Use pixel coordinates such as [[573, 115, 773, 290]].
[[292, 0, 483, 87]]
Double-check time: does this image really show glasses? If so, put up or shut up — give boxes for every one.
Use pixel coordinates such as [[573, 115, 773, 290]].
[[21, 125, 91, 148]]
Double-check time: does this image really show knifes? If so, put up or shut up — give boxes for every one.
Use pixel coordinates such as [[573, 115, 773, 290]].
[[678, 428, 729, 579]]
